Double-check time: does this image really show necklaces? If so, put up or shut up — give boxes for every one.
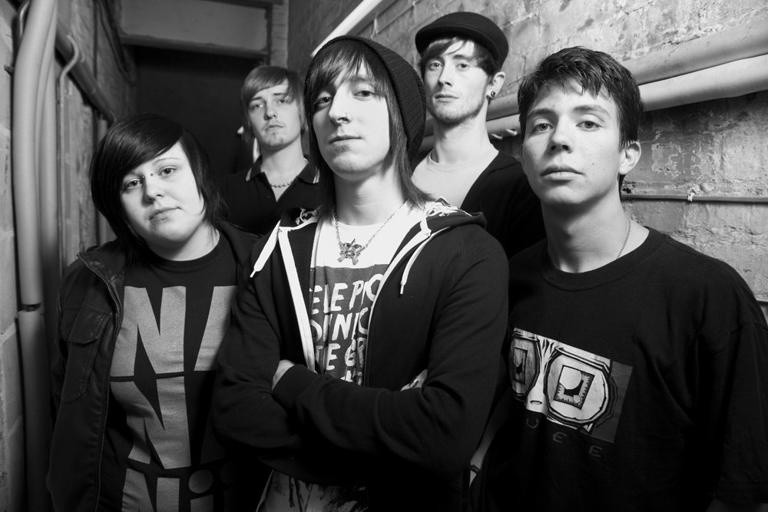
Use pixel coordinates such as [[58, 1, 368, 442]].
[[617, 216, 632, 257], [332, 192, 410, 265], [269, 182, 292, 189]]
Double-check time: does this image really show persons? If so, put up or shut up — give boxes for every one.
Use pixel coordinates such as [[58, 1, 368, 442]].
[[412, 12, 544, 254], [467, 46, 768, 512], [211, 33, 506, 512], [40, 113, 266, 512], [218, 63, 337, 237]]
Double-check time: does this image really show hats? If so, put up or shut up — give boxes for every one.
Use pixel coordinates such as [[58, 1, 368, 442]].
[[414, 11, 508, 68], [302, 35, 426, 160]]
